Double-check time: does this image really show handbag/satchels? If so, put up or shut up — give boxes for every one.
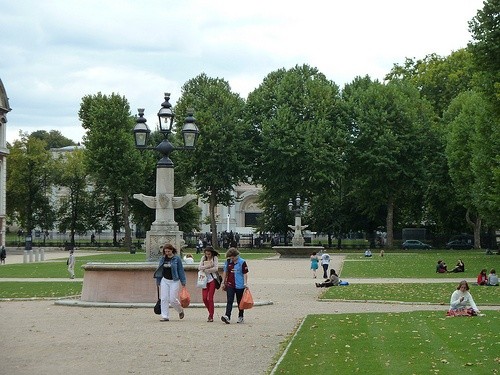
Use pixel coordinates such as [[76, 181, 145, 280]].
[[154, 299, 162, 314], [213, 276, 223, 289], [239, 288, 254, 310], [340, 280, 349, 285], [179, 288, 190, 307], [222, 279, 227, 291], [197, 271, 207, 289]]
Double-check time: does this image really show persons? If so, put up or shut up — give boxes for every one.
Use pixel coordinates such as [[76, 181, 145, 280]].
[[253, 231, 277, 248], [67, 250, 76, 278], [311, 248, 339, 290], [153, 243, 187, 321], [477, 269, 489, 286], [64, 240, 74, 250], [91, 234, 95, 244], [449, 258, 465, 273], [449, 280, 483, 318], [0, 245, 6, 264], [221, 247, 250, 324], [436, 260, 447, 273], [486, 269, 499, 286], [365, 249, 372, 256], [377, 234, 385, 249], [485, 247, 500, 255], [197, 246, 220, 322], [195, 229, 240, 254], [379, 249, 384, 257]]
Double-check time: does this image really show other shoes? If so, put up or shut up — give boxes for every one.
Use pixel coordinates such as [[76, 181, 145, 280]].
[[208, 317, 213, 322], [160, 318, 169, 322], [180, 310, 184, 319], [315, 283, 320, 287]]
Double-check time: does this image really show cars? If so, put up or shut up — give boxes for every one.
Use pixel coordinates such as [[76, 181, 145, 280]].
[[400, 240, 433, 250], [445, 240, 474, 250]]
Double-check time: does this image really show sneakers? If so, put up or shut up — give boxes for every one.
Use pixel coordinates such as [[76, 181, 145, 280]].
[[237, 317, 244, 323], [221, 316, 230, 324]]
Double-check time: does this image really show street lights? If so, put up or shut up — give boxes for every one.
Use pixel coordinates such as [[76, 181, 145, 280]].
[[288, 192, 310, 247], [132, 92, 200, 264]]
